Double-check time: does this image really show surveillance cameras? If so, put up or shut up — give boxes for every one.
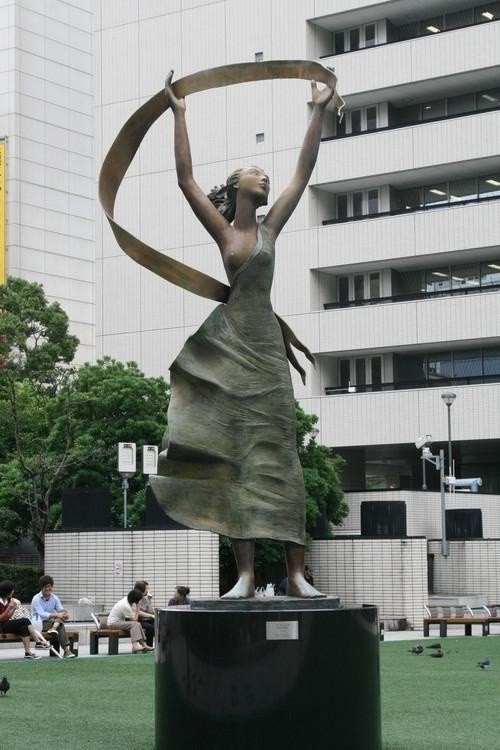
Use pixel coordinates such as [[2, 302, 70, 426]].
[[414, 435, 432, 449]]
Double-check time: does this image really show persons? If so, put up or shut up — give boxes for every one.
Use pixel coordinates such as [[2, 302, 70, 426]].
[[168, 586, 193, 607], [134, 581, 155, 647], [0, 580, 53, 660], [139, 65, 334, 598], [30, 575, 76, 659], [279, 562, 314, 595], [106, 588, 155, 653]]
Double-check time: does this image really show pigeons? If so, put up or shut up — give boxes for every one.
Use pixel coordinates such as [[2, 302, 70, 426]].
[[408, 644, 423, 655], [426, 642, 442, 649], [0, 675, 10, 695], [428, 649, 444, 658], [476, 656, 490, 669]]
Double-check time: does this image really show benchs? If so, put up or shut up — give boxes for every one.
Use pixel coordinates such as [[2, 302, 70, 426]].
[[0, 606, 137, 657], [423, 603, 500, 638]]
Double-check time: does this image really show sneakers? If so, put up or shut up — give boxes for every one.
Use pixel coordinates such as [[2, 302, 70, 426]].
[[47, 628, 58, 636], [132, 645, 154, 653], [63, 650, 76, 658], [35, 642, 53, 650], [24, 652, 42, 659]]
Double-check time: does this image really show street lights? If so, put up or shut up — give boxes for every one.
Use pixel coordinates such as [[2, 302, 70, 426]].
[[441, 392, 457, 477]]
